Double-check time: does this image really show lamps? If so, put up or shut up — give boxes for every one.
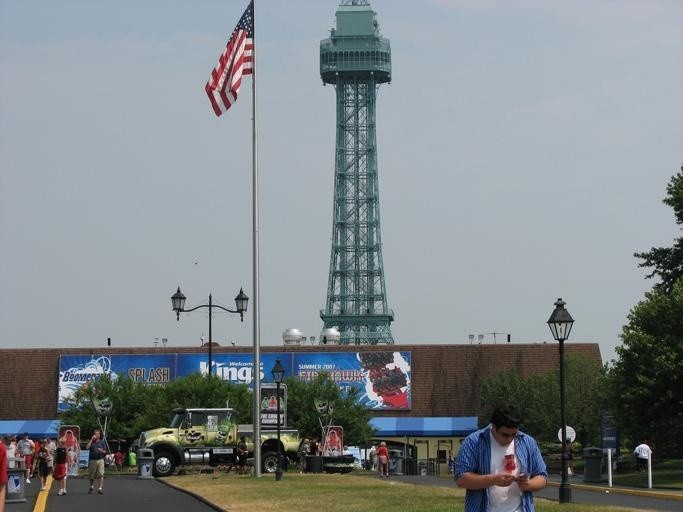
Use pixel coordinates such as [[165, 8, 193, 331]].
[[153, 338, 159, 348], [301, 337, 306, 346], [309, 335, 315, 346], [468, 334, 474, 345], [478, 335, 483, 345], [161, 338, 167, 348]]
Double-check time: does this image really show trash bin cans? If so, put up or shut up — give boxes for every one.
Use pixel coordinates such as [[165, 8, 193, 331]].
[[419, 462, 427, 477], [389, 450, 405, 476], [583, 447, 603, 483], [136, 448, 155, 480], [5, 469, 27, 503], [307, 456, 323, 473]]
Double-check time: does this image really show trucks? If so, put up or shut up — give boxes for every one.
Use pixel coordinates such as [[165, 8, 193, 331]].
[[137, 407, 301, 477]]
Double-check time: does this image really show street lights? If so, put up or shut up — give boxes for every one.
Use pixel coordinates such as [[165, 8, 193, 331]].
[[272, 359, 284, 481], [169, 284, 249, 383], [545, 298, 574, 502]]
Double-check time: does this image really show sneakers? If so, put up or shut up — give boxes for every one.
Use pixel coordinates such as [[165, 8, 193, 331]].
[[26, 479, 103, 496], [380, 476, 390, 478]]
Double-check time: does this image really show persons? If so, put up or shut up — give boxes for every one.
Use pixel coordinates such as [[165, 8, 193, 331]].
[[302, 438, 322, 457], [105, 451, 115, 467], [238, 436, 249, 475], [0, 429, 107, 512], [129, 451, 136, 468], [377, 442, 390, 477], [112, 450, 123, 471], [454, 405, 548, 512], [634, 439, 652, 472]]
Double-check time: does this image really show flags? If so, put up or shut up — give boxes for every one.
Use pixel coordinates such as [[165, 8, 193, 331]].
[[206, 1, 253, 117]]
